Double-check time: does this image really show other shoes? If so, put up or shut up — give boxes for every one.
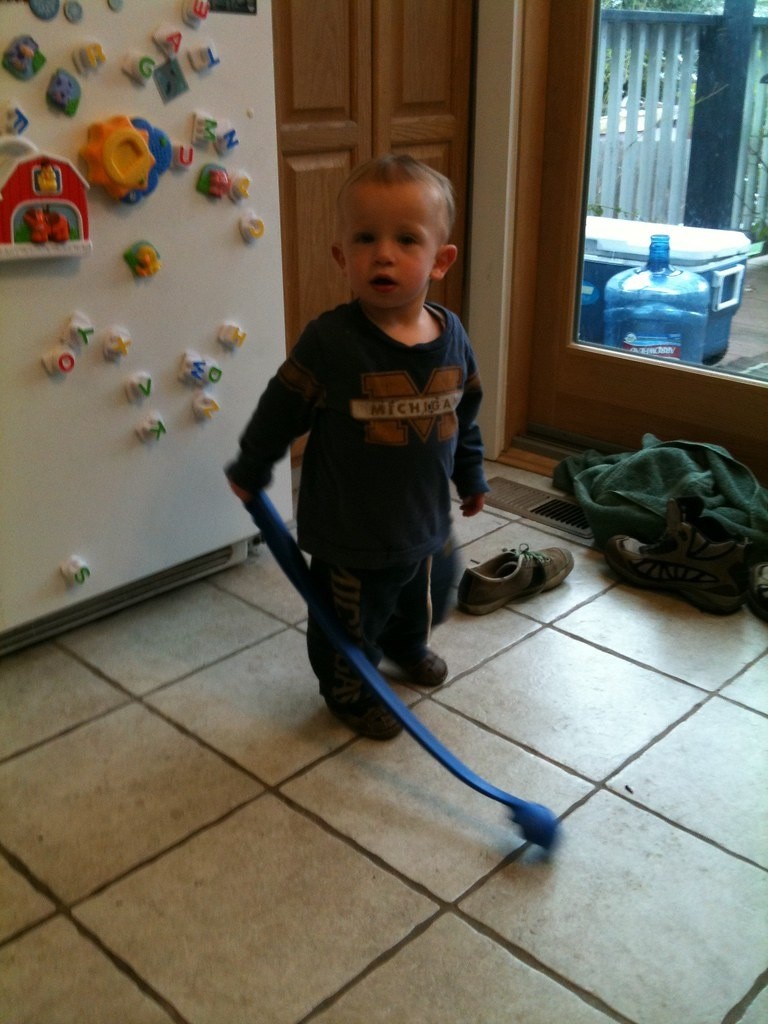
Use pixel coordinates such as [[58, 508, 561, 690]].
[[382, 647, 447, 685], [324, 699, 402, 739]]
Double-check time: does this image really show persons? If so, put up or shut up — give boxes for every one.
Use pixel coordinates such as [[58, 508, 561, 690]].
[[223, 152, 489, 740]]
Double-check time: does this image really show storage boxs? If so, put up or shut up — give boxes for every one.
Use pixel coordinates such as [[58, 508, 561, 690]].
[[580, 216, 751, 365]]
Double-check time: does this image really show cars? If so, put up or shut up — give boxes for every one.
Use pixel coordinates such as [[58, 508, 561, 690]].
[[599, 94, 678, 135]]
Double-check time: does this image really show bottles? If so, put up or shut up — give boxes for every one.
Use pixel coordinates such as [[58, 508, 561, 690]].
[[603, 233, 712, 363]]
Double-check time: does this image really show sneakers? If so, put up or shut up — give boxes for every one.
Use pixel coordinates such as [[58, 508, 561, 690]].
[[457, 543, 574, 616], [744, 562, 768, 622], [604, 494, 752, 614]]
